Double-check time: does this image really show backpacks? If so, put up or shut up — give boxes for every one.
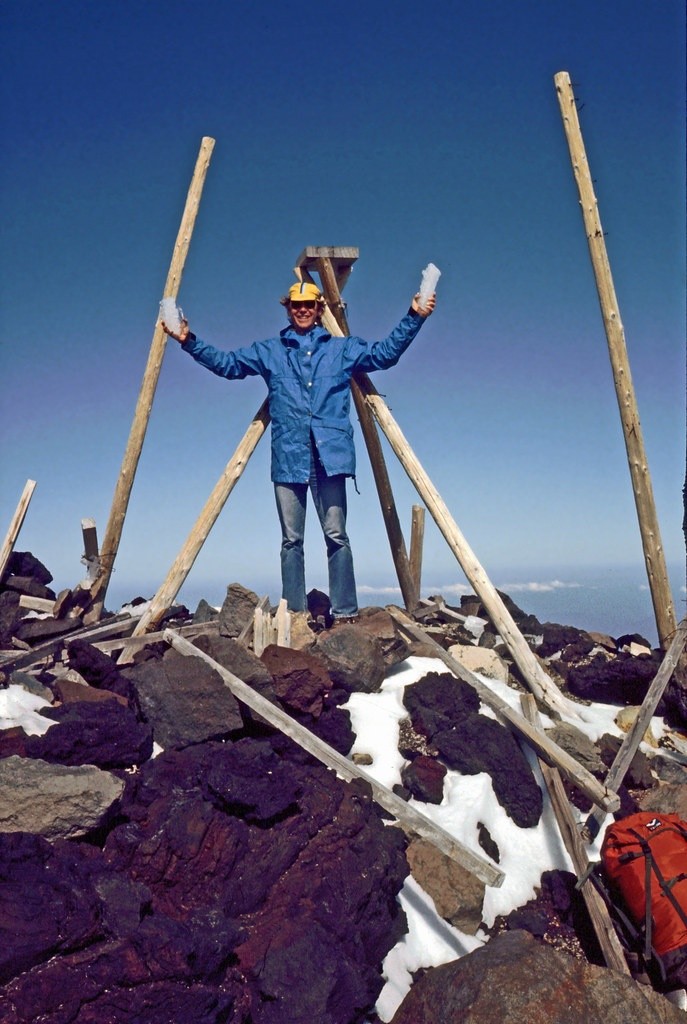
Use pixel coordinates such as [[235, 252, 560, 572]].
[[600, 811, 687, 993]]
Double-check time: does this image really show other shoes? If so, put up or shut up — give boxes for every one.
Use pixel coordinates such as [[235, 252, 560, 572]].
[[331, 617, 355, 628]]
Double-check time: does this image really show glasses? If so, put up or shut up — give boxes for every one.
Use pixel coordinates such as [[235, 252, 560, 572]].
[[291, 300, 315, 309]]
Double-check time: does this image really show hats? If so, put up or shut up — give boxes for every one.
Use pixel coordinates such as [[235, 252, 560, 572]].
[[289, 282, 321, 301]]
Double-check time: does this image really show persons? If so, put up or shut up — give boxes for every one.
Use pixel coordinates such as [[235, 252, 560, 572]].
[[159, 280, 436, 623]]
